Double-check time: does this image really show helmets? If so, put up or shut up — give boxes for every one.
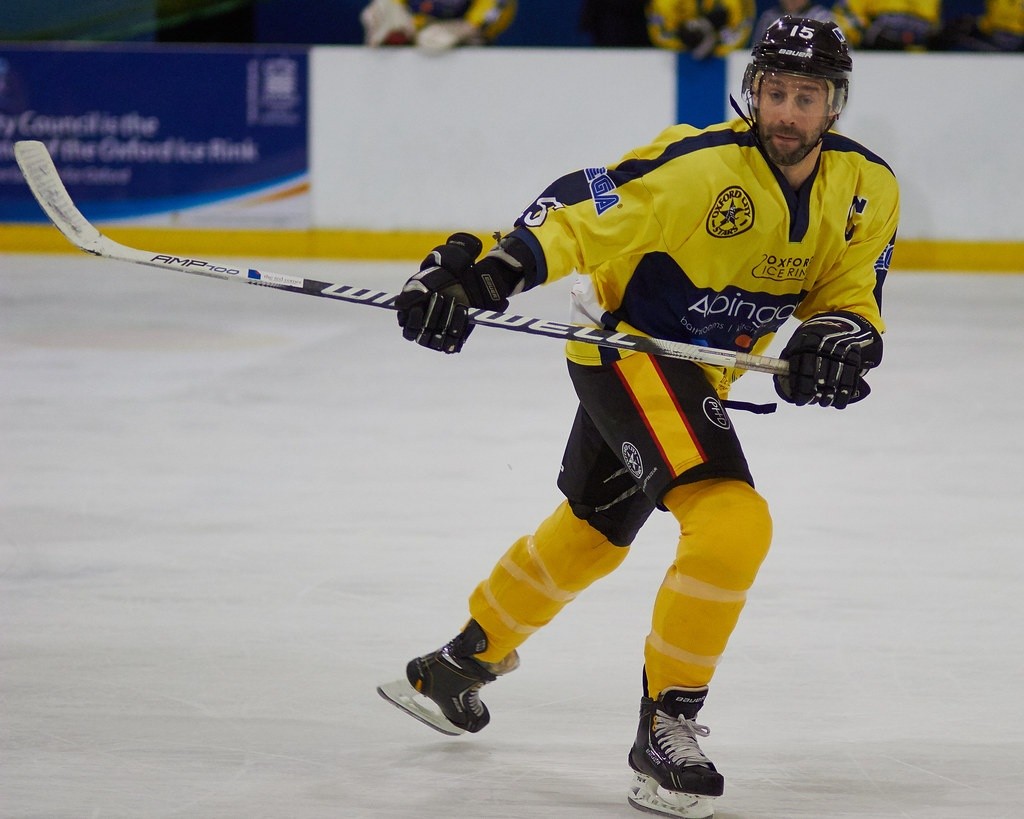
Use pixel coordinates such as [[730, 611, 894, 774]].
[[741, 14, 852, 115]]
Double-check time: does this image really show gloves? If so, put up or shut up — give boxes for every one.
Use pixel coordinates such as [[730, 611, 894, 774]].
[[397, 232, 518, 354], [773, 309, 883, 410]]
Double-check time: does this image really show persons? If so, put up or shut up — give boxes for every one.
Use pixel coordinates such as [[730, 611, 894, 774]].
[[643, 0, 1023, 57], [359, 0, 518, 51], [370, 15, 900, 819]]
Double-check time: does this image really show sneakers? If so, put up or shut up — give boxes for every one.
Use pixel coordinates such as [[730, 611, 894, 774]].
[[628, 663, 724, 818], [377, 617, 519, 737]]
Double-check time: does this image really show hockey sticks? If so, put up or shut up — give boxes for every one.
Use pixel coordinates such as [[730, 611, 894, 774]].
[[12, 140, 793, 375]]
